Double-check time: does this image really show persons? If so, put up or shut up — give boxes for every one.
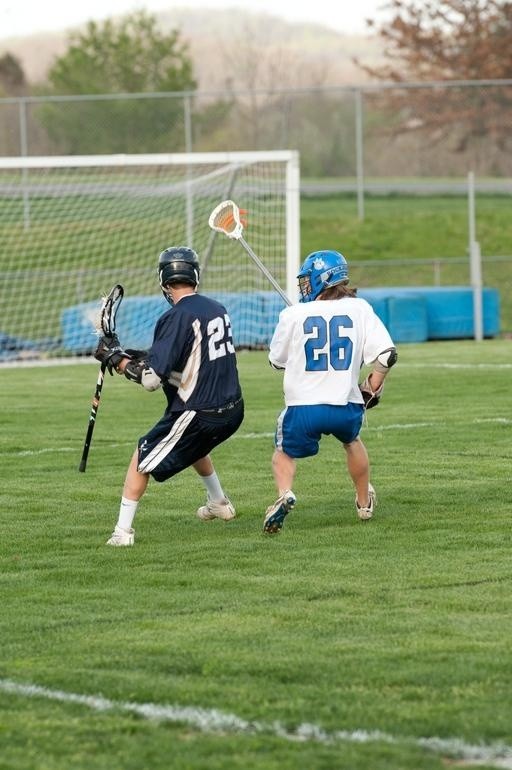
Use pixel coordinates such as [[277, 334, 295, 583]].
[[92, 245, 245, 547], [261, 250, 398, 534]]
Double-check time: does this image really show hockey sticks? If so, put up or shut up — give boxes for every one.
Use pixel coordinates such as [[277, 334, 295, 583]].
[[79, 284, 124, 473], [208, 199, 293, 305]]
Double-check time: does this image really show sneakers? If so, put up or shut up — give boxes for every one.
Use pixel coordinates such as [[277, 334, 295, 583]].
[[104, 522, 136, 547], [262, 488, 297, 535], [354, 479, 377, 521], [197, 496, 236, 522]]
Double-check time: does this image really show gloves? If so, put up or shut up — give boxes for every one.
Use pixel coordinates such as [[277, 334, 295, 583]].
[[94, 333, 127, 373], [124, 348, 150, 362]]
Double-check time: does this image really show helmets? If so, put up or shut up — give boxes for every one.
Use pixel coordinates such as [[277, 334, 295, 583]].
[[158, 246, 201, 307], [297, 249, 350, 303]]
